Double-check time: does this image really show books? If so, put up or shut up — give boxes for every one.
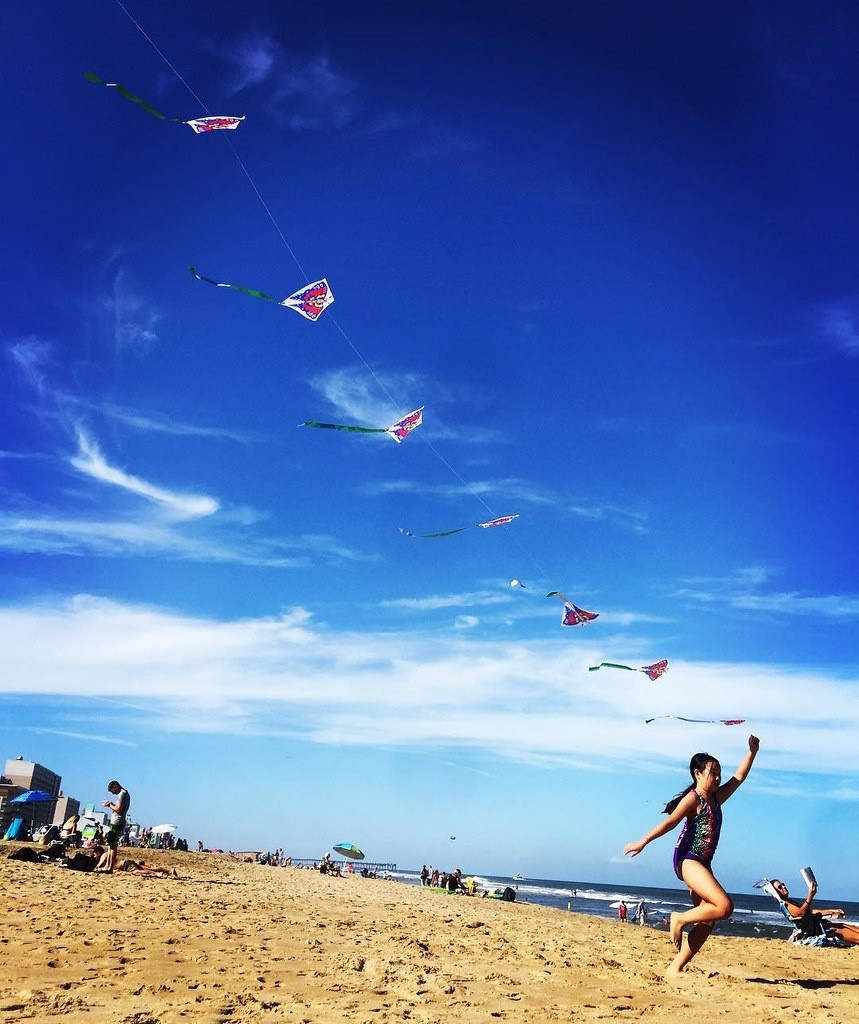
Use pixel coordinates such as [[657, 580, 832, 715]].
[[800, 866, 818, 888]]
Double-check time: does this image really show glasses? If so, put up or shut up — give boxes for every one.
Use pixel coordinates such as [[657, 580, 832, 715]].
[[777, 883, 785, 890]]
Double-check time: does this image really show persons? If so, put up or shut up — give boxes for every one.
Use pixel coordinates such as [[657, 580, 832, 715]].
[[38, 781, 188, 879], [254, 847, 292, 867], [420, 865, 501, 898], [295, 853, 395, 881], [568, 889, 577, 911], [197, 840, 203, 852], [229, 850, 240, 860], [663, 916, 669, 924], [729, 918, 738, 924], [623, 734, 760, 976], [770, 879, 859, 944], [619, 898, 647, 926]]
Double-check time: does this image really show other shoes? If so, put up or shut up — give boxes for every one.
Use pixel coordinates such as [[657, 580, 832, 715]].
[[96, 867, 113, 874]]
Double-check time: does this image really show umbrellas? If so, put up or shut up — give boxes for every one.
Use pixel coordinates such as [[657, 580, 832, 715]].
[[9, 790, 58, 837], [333, 843, 365, 876], [151, 824, 178, 849]]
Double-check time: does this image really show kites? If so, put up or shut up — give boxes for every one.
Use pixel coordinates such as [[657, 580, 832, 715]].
[[87, 66, 246, 135], [296, 404, 425, 443], [646, 715, 746, 726], [184, 264, 335, 323], [397, 514, 520, 540], [511, 578, 600, 627], [589, 659, 670, 682]]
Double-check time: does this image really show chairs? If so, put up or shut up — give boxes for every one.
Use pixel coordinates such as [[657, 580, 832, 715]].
[[753, 878, 842, 946]]
